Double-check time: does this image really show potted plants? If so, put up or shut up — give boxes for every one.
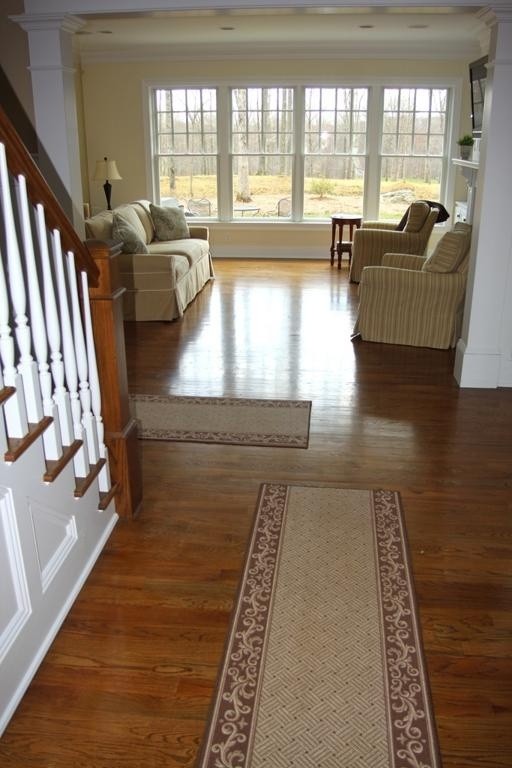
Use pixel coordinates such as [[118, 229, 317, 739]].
[[456, 133, 475, 160]]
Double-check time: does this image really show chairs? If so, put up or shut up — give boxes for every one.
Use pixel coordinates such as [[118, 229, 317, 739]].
[[277, 196, 291, 218], [188, 198, 211, 217], [348, 206, 440, 283], [357, 249, 469, 350]]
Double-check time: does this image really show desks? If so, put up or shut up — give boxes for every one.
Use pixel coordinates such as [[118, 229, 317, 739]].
[[234, 206, 260, 216]]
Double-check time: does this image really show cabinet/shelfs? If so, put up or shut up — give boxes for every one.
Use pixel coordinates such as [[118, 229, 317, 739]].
[[453, 207, 467, 224]]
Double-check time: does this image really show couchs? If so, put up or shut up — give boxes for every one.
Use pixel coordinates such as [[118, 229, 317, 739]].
[[118, 225, 214, 321]]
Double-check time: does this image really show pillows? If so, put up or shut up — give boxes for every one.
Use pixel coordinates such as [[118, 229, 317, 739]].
[[421, 221, 471, 273], [84, 199, 191, 255], [402, 201, 429, 232]]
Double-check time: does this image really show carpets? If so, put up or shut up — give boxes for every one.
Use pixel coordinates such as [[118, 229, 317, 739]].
[[194, 482, 442, 767], [128, 393, 312, 449]]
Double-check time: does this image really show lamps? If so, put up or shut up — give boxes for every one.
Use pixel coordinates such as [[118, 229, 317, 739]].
[[92, 156, 123, 209]]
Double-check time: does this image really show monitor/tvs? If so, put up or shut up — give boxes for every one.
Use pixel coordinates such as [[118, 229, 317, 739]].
[[468, 54, 489, 138]]
